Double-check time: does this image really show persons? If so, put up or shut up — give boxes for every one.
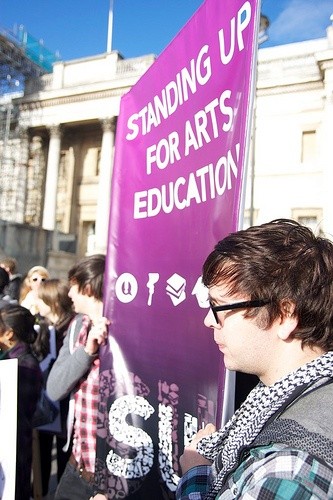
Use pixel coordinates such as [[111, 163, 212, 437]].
[[45, 253, 107, 500], [0, 258, 75, 500], [176, 218, 333, 500]]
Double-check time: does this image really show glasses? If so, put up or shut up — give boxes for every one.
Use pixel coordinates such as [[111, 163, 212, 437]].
[[209, 298, 276, 324], [32, 278, 45, 282]]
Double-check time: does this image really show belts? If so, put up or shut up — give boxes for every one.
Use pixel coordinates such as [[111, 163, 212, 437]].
[[70, 455, 95, 482]]
